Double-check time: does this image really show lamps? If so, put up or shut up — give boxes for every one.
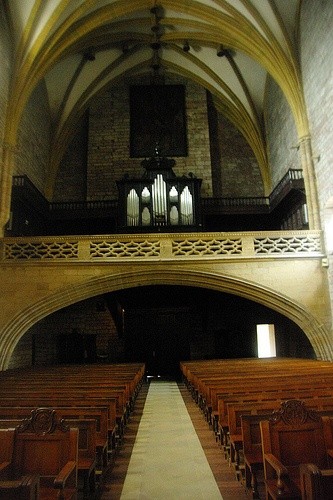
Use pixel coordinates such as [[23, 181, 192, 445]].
[[151, 57, 160, 68], [150, 4, 158, 12], [85, 47, 95, 59], [151, 40, 162, 51], [122, 43, 136, 51], [151, 24, 158, 32], [216, 45, 223, 57], [182, 40, 190, 52]]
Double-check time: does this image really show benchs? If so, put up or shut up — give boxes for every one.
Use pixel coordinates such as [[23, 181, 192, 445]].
[[0, 362, 144, 500], [181, 357, 333, 500]]
